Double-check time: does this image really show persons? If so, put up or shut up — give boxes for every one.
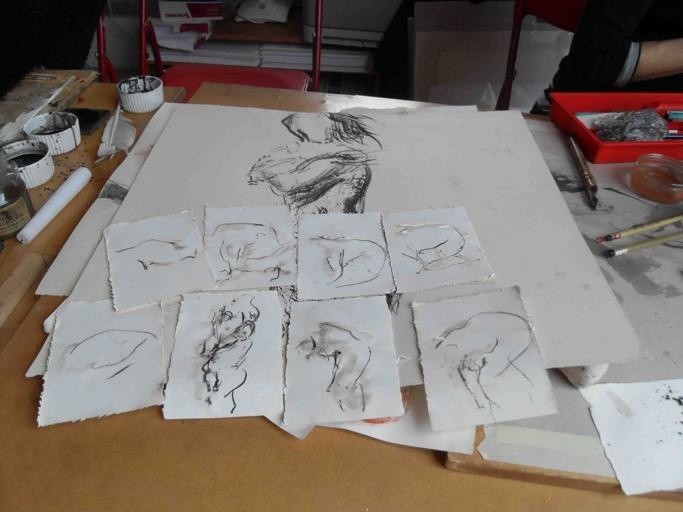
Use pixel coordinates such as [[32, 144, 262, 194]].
[[532, 0, 683, 112]]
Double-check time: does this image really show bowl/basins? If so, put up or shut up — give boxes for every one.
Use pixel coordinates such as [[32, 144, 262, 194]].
[[2, 111, 81, 191], [631, 152, 683, 204], [117, 77, 164, 113]]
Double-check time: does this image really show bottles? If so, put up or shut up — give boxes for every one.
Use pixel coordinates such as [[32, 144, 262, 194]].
[[0, 148, 36, 240]]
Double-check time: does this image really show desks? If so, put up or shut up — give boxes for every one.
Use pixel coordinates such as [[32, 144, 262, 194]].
[[4, 81, 682, 511], [2, 60, 191, 354]]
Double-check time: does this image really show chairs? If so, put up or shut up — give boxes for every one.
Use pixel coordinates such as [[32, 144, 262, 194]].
[[137, 2, 322, 102]]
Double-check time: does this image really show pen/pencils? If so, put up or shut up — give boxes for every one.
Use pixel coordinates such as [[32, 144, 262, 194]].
[[606, 216, 682, 256], [568, 135, 598, 208], [663, 110, 682, 140]]
